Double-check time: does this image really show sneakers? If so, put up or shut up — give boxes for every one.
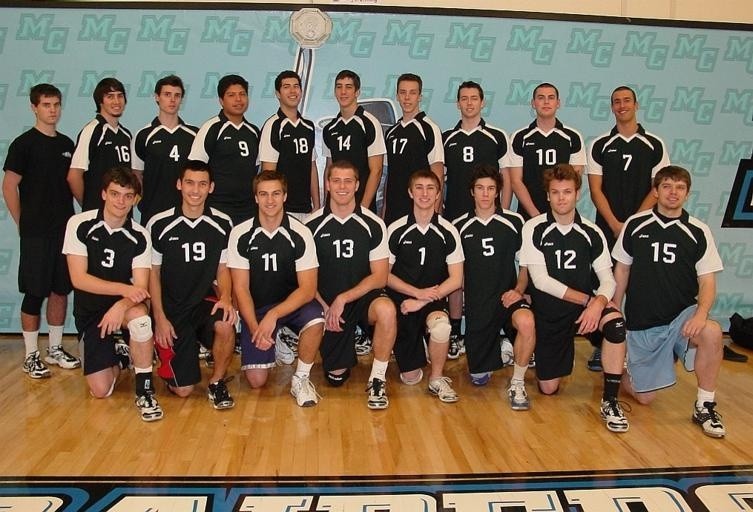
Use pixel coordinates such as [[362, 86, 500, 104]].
[[427, 376, 460, 403], [115, 337, 159, 369], [290, 372, 323, 408], [507, 378, 530, 410], [277, 329, 300, 358], [501, 337, 534, 368], [599, 397, 630, 433], [691, 399, 725, 439], [43, 344, 80, 370], [447, 334, 466, 360], [353, 333, 372, 356], [274, 334, 295, 365], [21, 350, 51, 379], [586, 348, 604, 372], [135, 391, 163, 421], [207, 375, 236, 411], [365, 376, 389, 410], [199, 345, 212, 360]]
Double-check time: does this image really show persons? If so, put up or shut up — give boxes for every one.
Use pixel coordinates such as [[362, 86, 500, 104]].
[[1, 70, 725, 438]]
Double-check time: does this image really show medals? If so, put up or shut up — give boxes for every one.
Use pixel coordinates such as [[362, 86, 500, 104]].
[[289, 7, 334, 48]]
